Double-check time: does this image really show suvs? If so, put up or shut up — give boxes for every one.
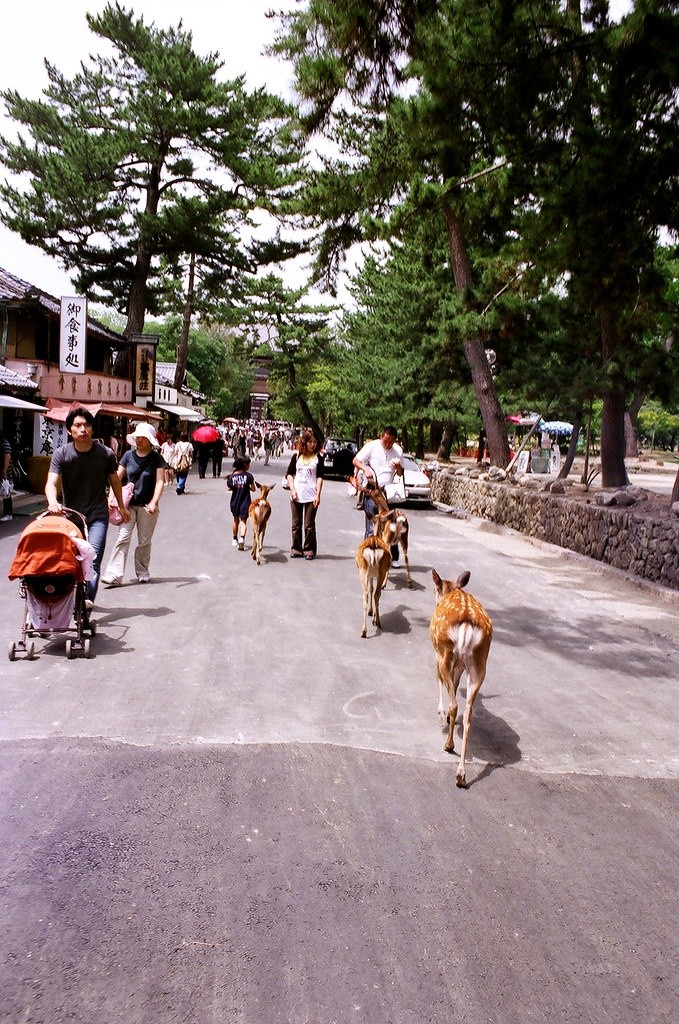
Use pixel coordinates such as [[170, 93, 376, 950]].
[[319, 437, 360, 479]]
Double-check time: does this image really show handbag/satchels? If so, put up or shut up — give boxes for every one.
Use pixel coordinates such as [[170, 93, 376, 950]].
[[109, 482, 135, 526], [281, 475, 290, 490], [386, 470, 406, 503]]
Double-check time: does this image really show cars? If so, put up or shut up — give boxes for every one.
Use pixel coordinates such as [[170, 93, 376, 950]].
[[403, 454, 431, 509]]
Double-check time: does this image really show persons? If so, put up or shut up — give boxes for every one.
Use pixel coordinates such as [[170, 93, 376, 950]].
[[224, 455, 256, 551], [351, 426, 405, 568], [284, 429, 324, 561], [156, 417, 318, 496], [45, 407, 132, 630], [100, 423, 169, 588], [0, 427, 15, 523]]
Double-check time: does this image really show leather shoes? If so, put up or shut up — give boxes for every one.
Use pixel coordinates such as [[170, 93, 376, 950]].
[[305, 551, 314, 559], [291, 549, 301, 557]]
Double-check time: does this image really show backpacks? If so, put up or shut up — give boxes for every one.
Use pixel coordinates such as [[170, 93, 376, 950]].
[[178, 442, 189, 472]]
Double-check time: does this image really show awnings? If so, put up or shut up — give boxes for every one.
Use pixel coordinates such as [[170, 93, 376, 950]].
[[147, 401, 203, 422], [0, 394, 50, 411], [40, 395, 165, 423]]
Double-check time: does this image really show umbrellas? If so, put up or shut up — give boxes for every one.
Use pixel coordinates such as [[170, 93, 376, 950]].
[[504, 410, 575, 454], [223, 418, 239, 425], [191, 425, 220, 444]]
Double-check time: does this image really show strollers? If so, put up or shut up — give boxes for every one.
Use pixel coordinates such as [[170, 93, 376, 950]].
[[8, 507, 97, 662]]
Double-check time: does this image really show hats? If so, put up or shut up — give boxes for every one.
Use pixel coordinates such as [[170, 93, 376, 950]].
[[127, 423, 160, 448]]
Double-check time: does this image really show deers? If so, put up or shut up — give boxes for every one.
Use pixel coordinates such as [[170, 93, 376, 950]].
[[356, 484, 414, 589], [430, 567, 494, 791], [247, 481, 277, 566], [354, 506, 396, 638]]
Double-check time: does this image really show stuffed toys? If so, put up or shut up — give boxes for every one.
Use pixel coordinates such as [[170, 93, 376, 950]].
[[72, 537, 98, 582]]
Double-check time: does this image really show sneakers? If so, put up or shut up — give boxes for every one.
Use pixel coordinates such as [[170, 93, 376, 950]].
[[232, 539, 237, 547], [238, 538, 244, 550], [1, 515, 13, 521], [391, 561, 401, 568], [138, 576, 150, 583], [101, 576, 122, 586]]
